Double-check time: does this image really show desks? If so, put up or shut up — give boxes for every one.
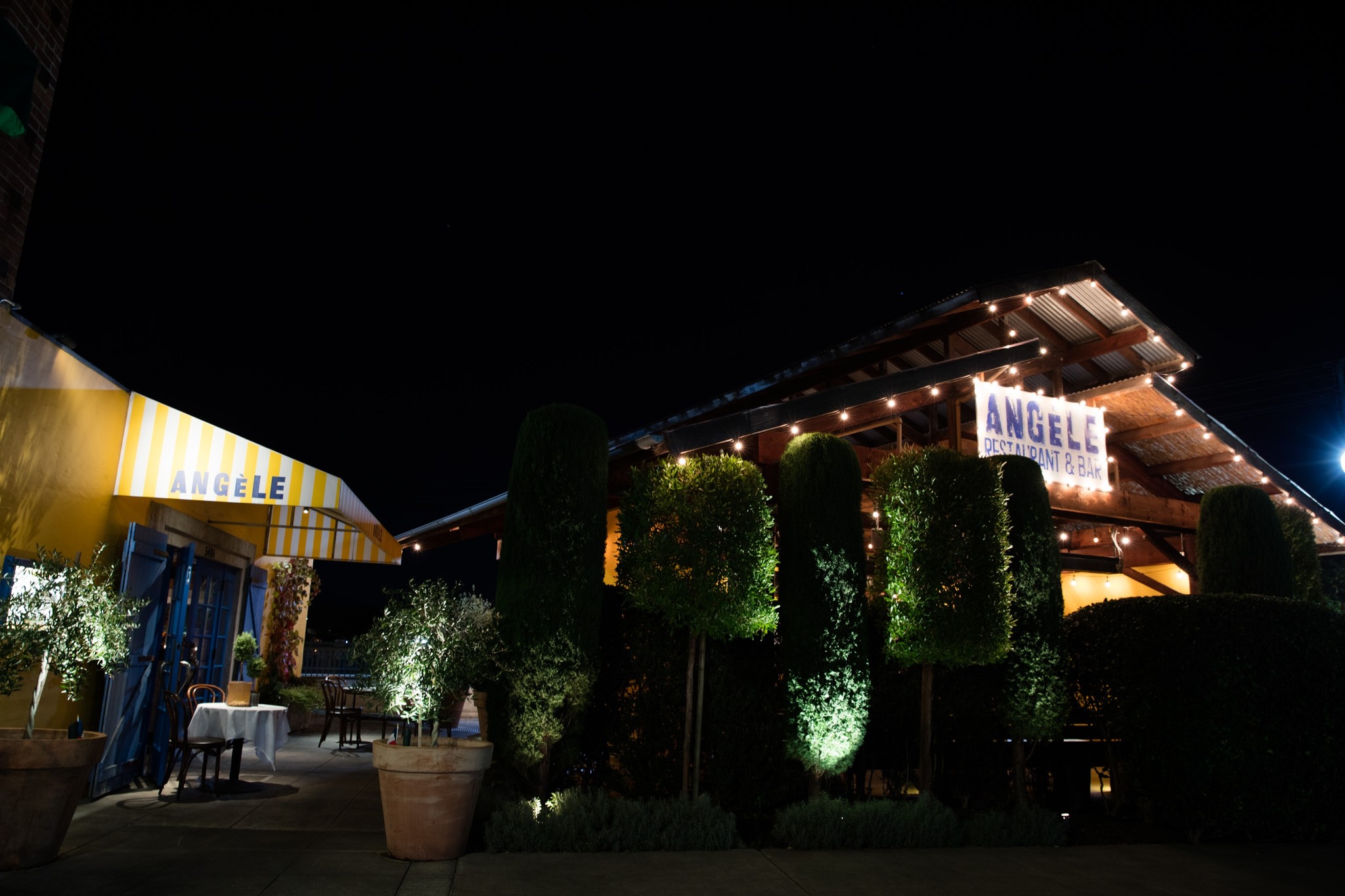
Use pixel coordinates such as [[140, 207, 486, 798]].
[[342, 685, 389, 739], [198, 701, 289, 793]]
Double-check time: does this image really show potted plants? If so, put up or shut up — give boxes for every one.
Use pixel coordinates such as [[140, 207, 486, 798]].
[[227, 629, 258, 708], [245, 655, 269, 706], [348, 574, 518, 861], [0, 538, 154, 870]]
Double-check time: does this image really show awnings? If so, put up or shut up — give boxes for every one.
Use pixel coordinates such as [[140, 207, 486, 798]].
[[113, 391, 403, 566]]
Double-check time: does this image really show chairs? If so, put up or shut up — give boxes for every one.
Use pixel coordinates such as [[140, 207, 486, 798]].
[[158, 675, 363, 801]]
